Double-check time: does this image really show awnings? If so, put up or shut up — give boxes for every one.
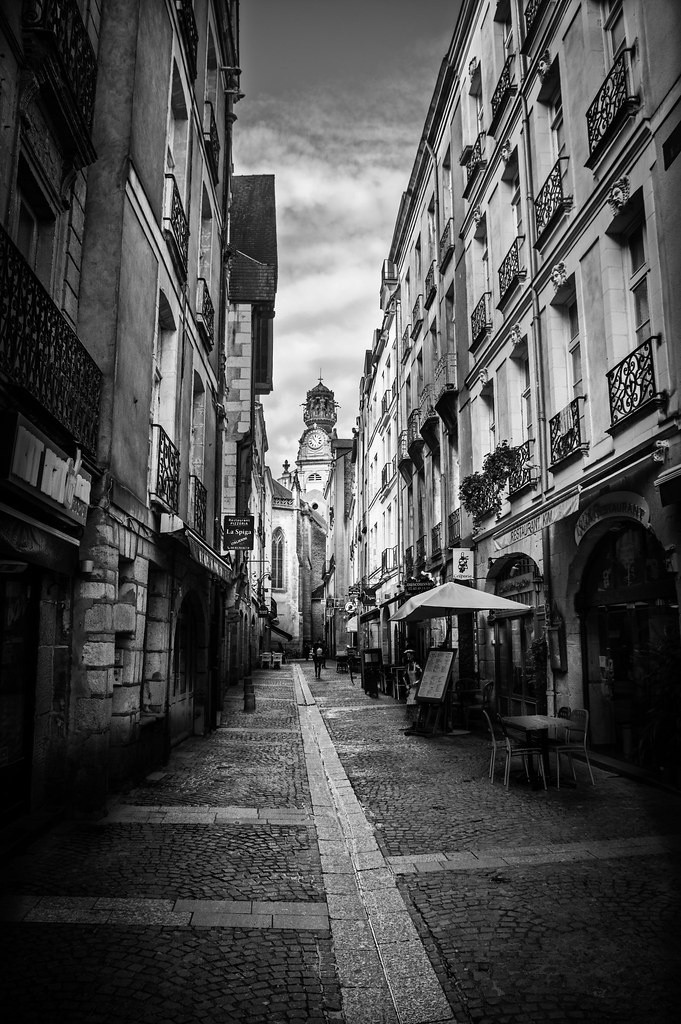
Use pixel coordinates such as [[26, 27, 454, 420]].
[[492, 440, 664, 552], [346, 607, 381, 632]]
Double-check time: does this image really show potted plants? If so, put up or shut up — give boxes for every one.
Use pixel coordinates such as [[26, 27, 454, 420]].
[[458, 436, 521, 529]]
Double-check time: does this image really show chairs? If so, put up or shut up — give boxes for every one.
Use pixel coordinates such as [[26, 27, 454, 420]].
[[482, 706, 597, 790], [380, 665, 407, 701], [448, 678, 495, 730], [259, 652, 283, 669]]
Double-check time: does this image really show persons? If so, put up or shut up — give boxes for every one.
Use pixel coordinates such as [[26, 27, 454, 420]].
[[403, 649, 422, 720], [304, 637, 327, 679]]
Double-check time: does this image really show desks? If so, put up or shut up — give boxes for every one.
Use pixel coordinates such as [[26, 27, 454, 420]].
[[451, 689, 481, 731], [499, 715, 577, 791]]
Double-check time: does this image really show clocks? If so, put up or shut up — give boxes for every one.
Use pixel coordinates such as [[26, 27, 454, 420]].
[[308, 432, 324, 450]]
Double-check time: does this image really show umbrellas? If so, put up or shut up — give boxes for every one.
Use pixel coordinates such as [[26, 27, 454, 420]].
[[387, 581, 531, 647]]
